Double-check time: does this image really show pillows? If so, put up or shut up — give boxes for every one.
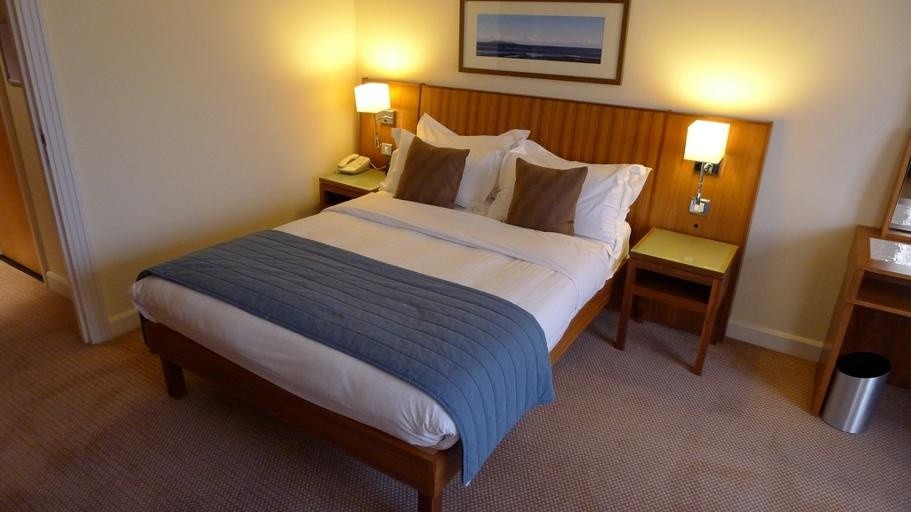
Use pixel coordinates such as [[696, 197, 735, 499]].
[[381, 111, 653, 258]]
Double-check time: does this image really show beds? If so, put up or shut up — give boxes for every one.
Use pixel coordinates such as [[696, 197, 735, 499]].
[[131, 78, 773, 512]]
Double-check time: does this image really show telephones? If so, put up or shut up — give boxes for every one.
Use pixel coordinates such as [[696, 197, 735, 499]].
[[337, 153, 370, 175]]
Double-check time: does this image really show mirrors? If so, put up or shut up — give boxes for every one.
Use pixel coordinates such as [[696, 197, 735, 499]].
[[880, 138, 910, 241]]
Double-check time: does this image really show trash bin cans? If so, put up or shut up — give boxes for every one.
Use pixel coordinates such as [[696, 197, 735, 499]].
[[821, 351, 893, 435]]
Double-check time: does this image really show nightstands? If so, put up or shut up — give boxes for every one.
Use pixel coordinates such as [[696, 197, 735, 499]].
[[616, 226, 740, 376], [319, 169, 388, 208]]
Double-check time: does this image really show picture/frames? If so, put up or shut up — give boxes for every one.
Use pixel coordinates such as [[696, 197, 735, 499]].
[[459, 1, 631, 86]]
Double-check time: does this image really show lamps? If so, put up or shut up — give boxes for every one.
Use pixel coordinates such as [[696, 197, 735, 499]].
[[684, 120, 730, 214], [354, 82, 391, 149]]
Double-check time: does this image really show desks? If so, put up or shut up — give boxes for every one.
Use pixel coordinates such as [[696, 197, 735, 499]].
[[810, 225, 911, 417]]
